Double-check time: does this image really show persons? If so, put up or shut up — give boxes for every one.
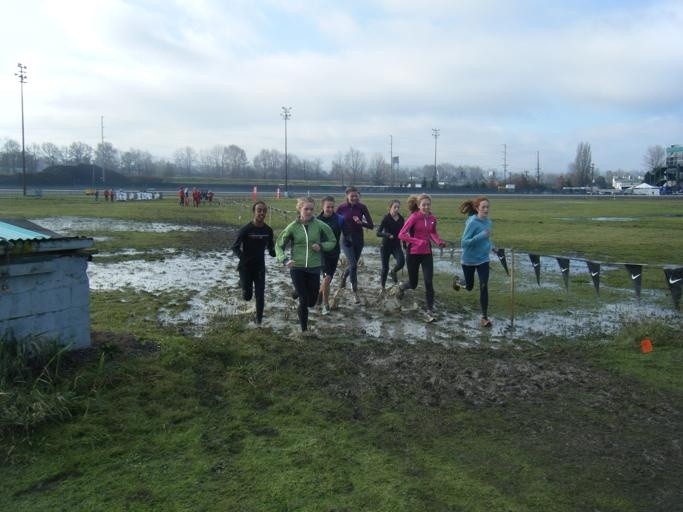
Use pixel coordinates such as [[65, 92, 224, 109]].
[[337, 188, 374, 293], [109, 188, 114, 202], [178, 186, 215, 209], [451, 196, 493, 328], [398, 191, 446, 322], [103, 189, 109, 202], [231, 202, 276, 324], [94, 188, 100, 202], [273, 198, 336, 330], [317, 196, 345, 316], [375, 199, 407, 287]]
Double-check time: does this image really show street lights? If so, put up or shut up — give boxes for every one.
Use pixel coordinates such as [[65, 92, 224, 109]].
[[278, 106, 292, 199], [13, 63, 27, 196], [430, 127, 439, 184]]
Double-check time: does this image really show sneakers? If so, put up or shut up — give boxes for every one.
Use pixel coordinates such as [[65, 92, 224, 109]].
[[422, 314, 436, 323], [352, 294, 361, 305], [395, 284, 404, 299], [321, 304, 331, 316], [479, 316, 491, 328], [452, 275, 460, 291]]
